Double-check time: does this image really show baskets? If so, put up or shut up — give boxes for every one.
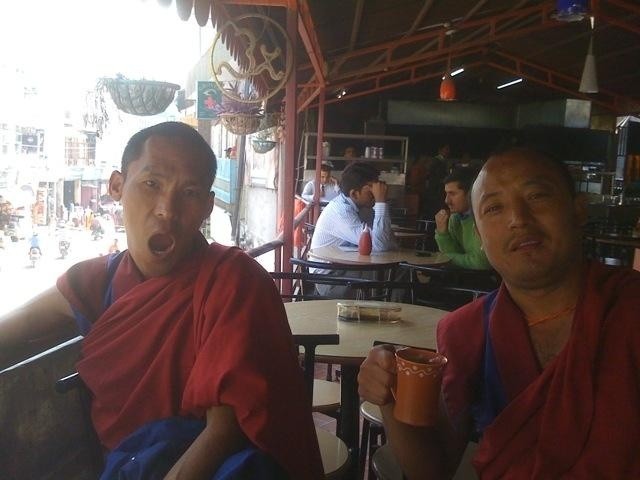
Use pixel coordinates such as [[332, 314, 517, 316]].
[[104, 77, 180, 116], [217, 113, 259, 136], [253, 140, 276, 153]]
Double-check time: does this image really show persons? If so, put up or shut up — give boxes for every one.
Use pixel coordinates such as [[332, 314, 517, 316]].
[[357, 144, 640, 480], [27, 194, 127, 258], [2, 121, 326, 478], [302, 146, 495, 306]]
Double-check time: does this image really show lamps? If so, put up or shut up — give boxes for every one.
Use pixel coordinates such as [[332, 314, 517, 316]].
[[439, 32, 456, 99], [579, 33, 599, 95], [337, 87, 346, 99]]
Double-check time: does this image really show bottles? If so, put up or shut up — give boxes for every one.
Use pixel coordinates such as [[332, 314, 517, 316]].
[[358, 223, 373, 257]]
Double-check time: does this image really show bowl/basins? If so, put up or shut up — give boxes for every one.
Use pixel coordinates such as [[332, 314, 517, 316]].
[[415, 219, 435, 232]]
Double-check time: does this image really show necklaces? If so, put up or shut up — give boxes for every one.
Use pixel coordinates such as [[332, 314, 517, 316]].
[[525, 306, 577, 328]]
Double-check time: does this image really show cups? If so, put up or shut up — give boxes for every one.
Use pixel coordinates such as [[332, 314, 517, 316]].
[[379, 167, 406, 185], [388, 346, 449, 429], [365, 145, 383, 160], [322, 141, 330, 158]]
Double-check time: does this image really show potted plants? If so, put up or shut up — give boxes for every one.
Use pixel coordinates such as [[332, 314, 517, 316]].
[[82, 72, 181, 140], [253, 127, 277, 153], [204, 77, 266, 136]]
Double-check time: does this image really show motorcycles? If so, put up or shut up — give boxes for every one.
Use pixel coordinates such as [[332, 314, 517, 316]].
[[28, 248, 41, 269], [58, 241, 69, 260]]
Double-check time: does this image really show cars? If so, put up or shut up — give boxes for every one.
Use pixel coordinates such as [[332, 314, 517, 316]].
[[101, 190, 114, 206]]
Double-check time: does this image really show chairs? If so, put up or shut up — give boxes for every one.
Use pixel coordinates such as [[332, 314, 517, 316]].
[[57, 331, 340, 479], [264, 271, 393, 410], [580, 214, 636, 269], [289, 257, 391, 301]]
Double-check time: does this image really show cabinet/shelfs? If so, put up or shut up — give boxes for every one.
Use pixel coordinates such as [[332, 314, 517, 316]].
[[304, 132, 406, 214]]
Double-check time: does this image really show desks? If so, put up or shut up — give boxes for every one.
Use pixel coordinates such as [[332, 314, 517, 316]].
[[282, 300, 454, 450], [308, 245, 451, 298], [392, 222, 425, 242]]
